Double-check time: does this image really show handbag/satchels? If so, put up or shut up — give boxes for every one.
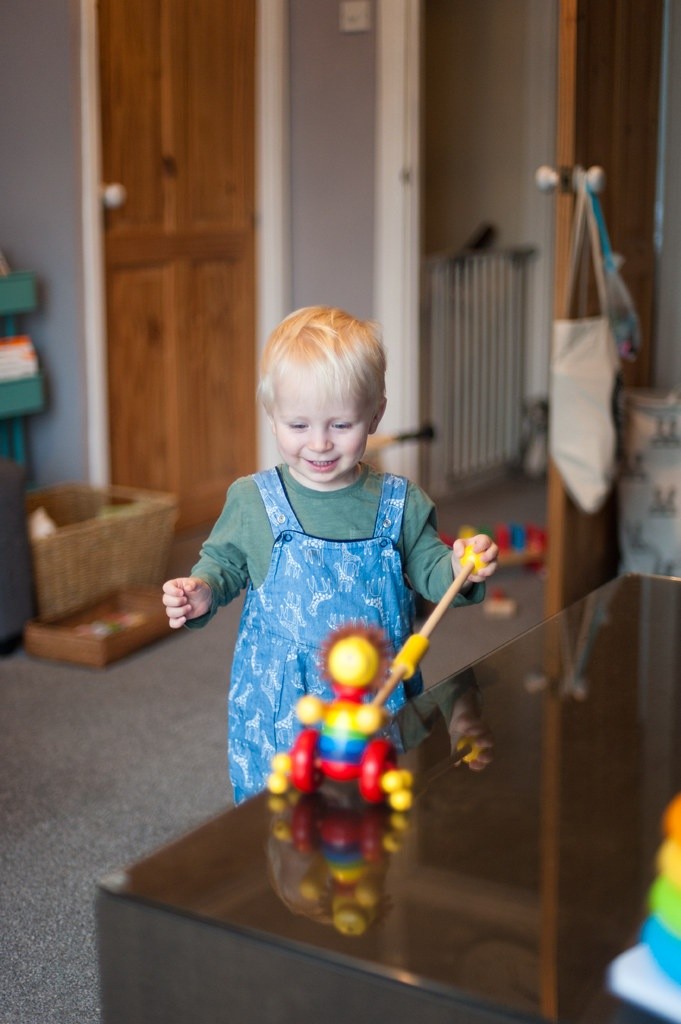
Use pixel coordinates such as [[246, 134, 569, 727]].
[[548, 174, 621, 513]]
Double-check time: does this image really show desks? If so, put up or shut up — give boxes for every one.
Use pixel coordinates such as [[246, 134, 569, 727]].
[[97, 570, 681, 1024]]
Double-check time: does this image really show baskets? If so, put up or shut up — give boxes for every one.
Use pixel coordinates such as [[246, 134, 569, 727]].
[[19, 480, 179, 623]]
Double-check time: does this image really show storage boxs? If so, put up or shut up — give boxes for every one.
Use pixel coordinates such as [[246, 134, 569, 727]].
[[28, 587, 169, 666]]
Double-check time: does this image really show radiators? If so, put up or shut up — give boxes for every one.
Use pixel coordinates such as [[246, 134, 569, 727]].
[[425, 245, 529, 495]]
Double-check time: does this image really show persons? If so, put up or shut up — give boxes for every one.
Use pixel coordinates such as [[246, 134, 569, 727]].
[[161, 303, 500, 807], [266, 667, 498, 925]]
